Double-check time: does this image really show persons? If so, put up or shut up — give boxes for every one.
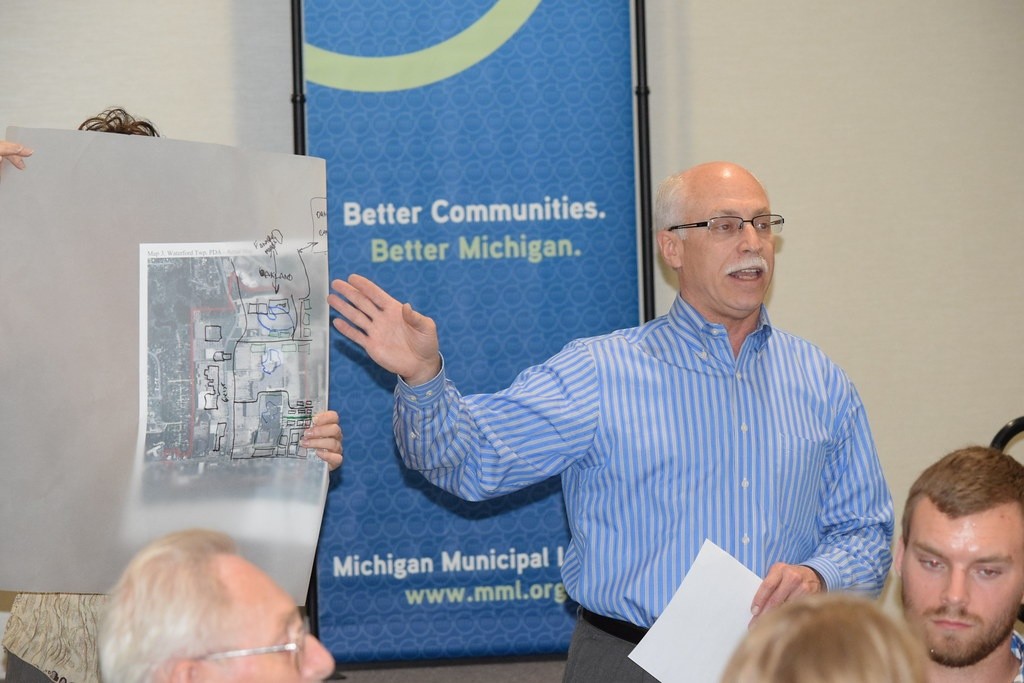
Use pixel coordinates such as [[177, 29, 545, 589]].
[[328, 161, 896, 683], [723, 591, 928, 683], [1, 109, 343, 683], [99, 529, 337, 683], [889, 446, 1024, 683]]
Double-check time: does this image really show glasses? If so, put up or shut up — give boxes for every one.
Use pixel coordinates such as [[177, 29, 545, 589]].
[[672, 214, 787, 238], [200, 618, 312, 673]]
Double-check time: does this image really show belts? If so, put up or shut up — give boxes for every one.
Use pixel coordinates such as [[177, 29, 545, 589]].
[[581, 608, 648, 644]]
